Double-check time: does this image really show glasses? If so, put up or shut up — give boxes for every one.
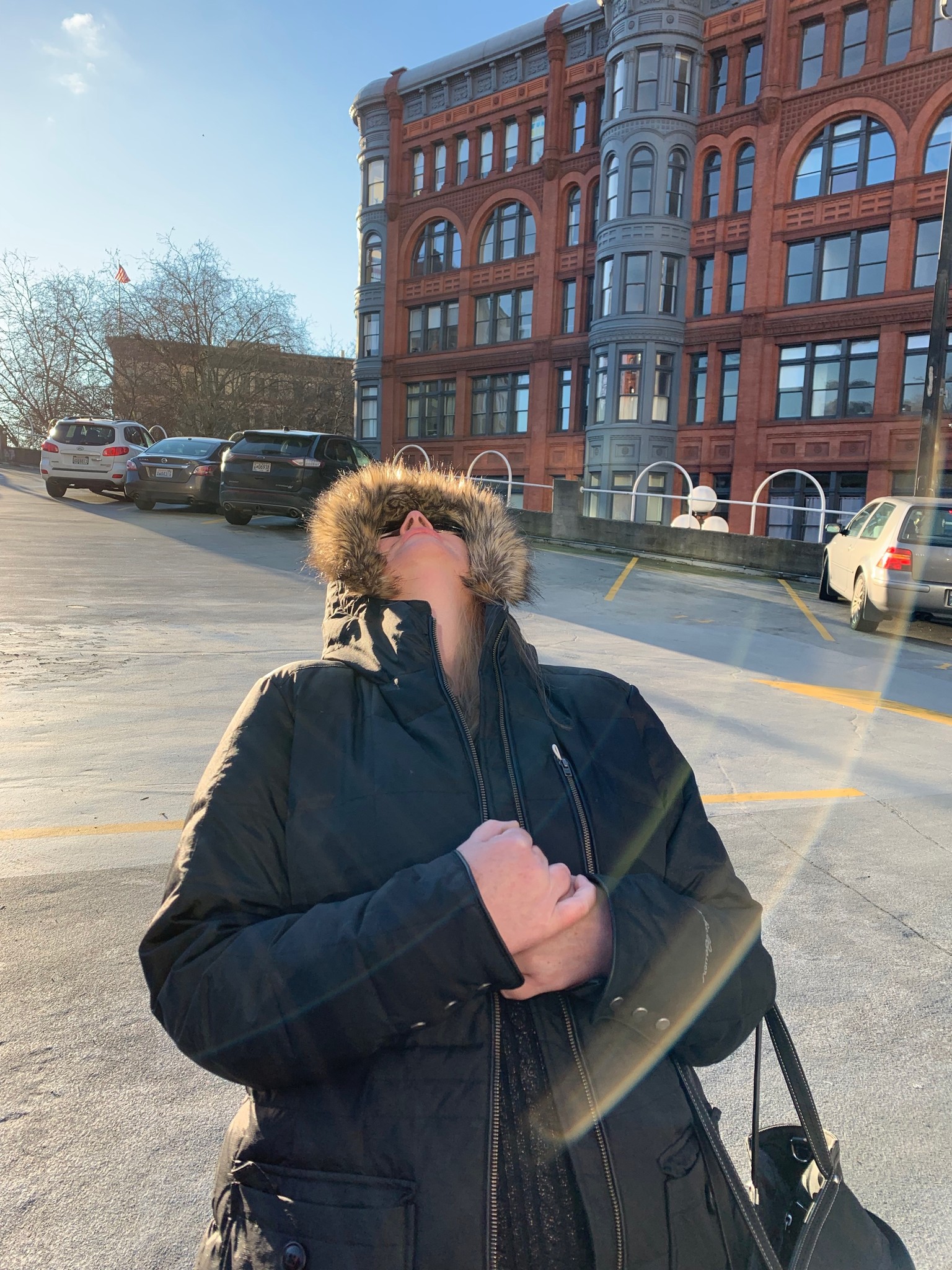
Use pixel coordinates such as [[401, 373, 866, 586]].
[[379, 522, 465, 538]]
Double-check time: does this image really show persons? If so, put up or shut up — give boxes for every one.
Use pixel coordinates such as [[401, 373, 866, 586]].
[[138, 456, 775, 1270]]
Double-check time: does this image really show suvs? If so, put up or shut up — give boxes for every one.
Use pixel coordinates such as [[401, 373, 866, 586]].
[[39, 415, 163, 503], [220, 426, 384, 526]]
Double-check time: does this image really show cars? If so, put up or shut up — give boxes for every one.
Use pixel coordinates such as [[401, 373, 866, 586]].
[[124, 436, 235, 514], [818, 496, 952, 634]]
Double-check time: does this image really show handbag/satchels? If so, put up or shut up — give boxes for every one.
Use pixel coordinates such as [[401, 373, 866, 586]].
[[686, 905, 915, 1270]]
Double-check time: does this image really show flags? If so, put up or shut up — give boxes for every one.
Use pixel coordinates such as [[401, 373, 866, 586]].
[[115, 263, 131, 283]]
